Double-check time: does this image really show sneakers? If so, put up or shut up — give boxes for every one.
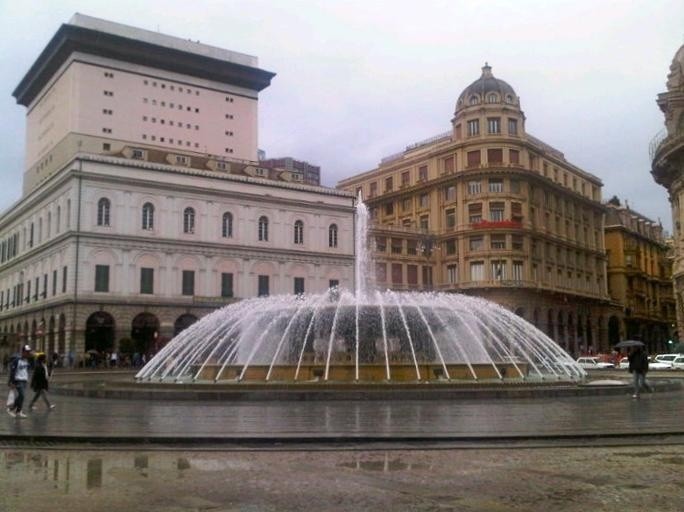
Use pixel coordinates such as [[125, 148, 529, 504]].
[[16, 410, 26, 418], [7, 408, 17, 418]]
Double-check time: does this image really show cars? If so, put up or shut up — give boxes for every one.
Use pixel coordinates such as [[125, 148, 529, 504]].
[[540, 352, 684, 371]]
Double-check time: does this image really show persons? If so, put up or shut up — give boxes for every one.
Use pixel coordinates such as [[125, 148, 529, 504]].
[[7, 343, 32, 419], [311, 332, 403, 363], [67, 349, 153, 370], [625, 346, 654, 398], [29, 354, 57, 410]]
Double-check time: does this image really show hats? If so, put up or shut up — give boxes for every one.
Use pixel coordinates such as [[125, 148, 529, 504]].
[[22, 345, 32, 352]]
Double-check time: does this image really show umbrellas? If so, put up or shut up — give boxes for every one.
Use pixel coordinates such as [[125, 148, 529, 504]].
[[614, 339, 644, 347]]
